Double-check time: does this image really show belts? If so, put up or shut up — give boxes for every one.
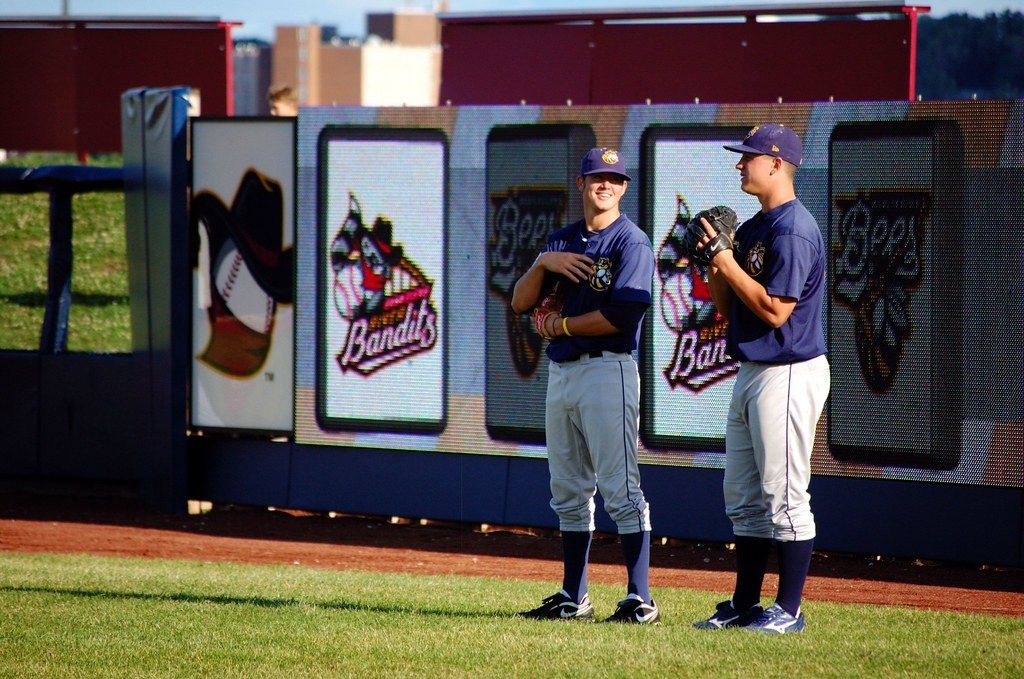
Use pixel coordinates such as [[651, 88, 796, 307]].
[[566, 351, 632, 362]]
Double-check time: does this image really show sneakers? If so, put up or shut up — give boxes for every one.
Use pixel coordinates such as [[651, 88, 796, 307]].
[[600, 593, 662, 625], [742, 602, 805, 637], [514, 589, 594, 621], [692, 600, 763, 632]]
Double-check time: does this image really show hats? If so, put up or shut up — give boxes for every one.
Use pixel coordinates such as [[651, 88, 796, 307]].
[[581, 146, 632, 182], [723, 124, 803, 167]]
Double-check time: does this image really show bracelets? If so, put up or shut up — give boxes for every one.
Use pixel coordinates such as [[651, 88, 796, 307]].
[[563, 318, 572, 336], [552, 317, 560, 337]]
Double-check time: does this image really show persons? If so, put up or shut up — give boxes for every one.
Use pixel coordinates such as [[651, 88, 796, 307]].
[[692, 124, 830, 634], [511, 147, 660, 628]]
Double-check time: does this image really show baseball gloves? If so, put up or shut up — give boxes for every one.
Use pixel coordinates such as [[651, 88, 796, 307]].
[[683, 204, 738, 263], [534, 294, 563, 341]]
